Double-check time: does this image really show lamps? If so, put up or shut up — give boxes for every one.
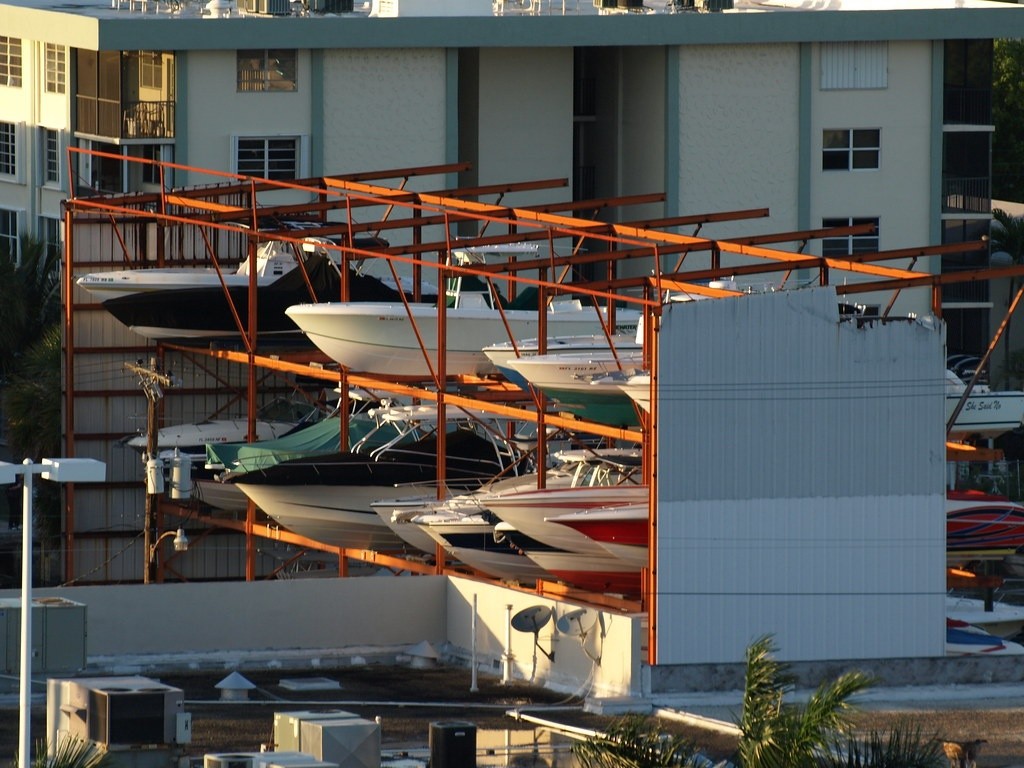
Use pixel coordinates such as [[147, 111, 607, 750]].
[[149, 528, 188, 563]]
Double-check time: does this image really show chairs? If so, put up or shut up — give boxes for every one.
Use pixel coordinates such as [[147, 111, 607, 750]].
[[123, 103, 166, 138]]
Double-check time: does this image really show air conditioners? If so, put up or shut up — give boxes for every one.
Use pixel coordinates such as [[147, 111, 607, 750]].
[[617, 0, 643, 8], [236, 0, 259, 13], [258, 0, 290, 14], [694, 0, 735, 11], [593, 0, 617, 8]]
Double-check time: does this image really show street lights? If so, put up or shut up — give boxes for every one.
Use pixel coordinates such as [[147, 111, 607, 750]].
[[0, 458, 107, 768], [144, 524, 190, 585]]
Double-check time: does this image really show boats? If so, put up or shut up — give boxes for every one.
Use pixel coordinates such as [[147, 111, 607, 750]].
[[75, 220, 1024, 657]]
